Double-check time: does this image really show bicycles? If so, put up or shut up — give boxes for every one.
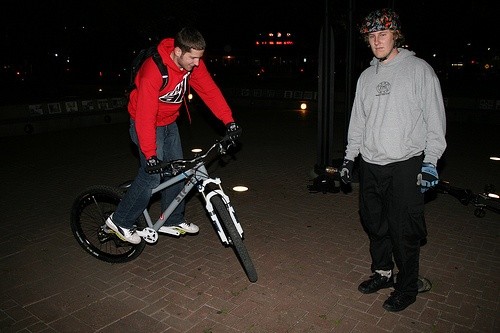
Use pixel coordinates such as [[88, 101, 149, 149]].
[[434, 176, 500, 219], [69, 126, 258, 283]]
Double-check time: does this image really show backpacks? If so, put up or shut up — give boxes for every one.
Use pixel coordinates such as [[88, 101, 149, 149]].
[[130, 46, 168, 87]]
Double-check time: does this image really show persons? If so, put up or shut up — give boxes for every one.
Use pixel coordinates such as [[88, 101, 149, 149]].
[[106, 25, 243, 244], [340, 8, 446, 312]]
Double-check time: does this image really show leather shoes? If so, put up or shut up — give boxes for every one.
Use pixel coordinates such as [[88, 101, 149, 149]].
[[358, 270, 394, 294], [383, 283, 416, 312]]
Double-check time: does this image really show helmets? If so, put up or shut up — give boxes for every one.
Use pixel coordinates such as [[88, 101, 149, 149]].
[[360, 8, 401, 34]]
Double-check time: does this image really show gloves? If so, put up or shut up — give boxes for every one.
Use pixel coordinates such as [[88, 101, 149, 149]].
[[225, 122, 242, 147], [339, 158, 354, 184], [414, 163, 438, 193], [146, 156, 164, 175]]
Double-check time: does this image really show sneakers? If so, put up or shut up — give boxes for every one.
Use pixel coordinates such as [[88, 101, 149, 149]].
[[104, 212, 141, 244], [178, 218, 199, 233]]
